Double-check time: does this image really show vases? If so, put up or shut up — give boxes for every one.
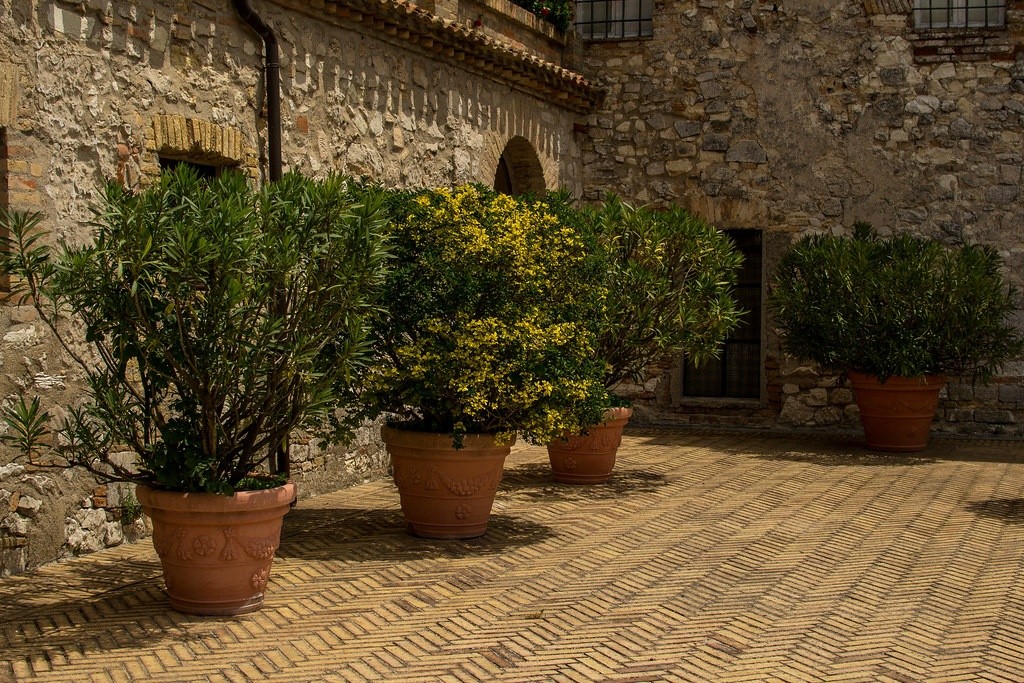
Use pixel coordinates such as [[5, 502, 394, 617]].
[[379, 423, 518, 541]]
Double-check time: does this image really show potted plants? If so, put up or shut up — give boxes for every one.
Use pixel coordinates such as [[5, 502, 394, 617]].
[[764, 220, 1024, 453], [0, 160, 401, 618], [514, 183, 752, 486]]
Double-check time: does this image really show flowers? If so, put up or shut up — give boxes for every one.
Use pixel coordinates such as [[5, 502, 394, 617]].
[[319, 176, 627, 451]]
